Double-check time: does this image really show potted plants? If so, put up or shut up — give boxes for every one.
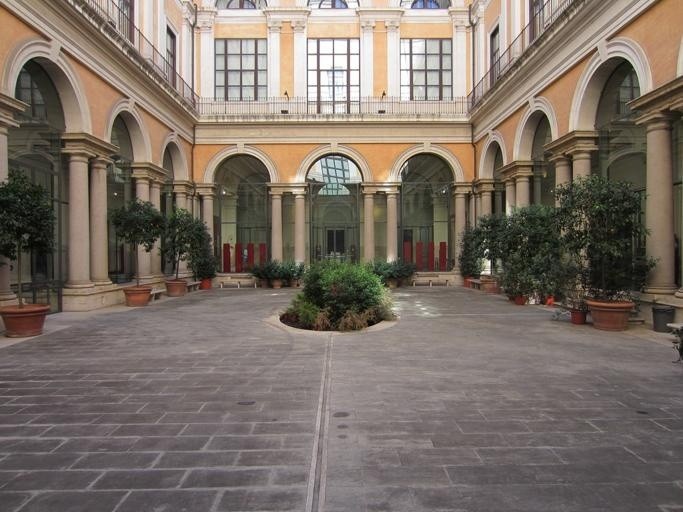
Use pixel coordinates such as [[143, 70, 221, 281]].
[[251, 266, 268, 288], [0, 163, 59, 339], [160, 204, 197, 299], [110, 196, 166, 308], [455, 175, 662, 335], [390, 260, 416, 287], [264, 258, 306, 290], [190, 230, 217, 290]]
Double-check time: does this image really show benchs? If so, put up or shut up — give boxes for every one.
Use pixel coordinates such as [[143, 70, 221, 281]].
[[216, 277, 260, 288], [410, 274, 454, 288]]
